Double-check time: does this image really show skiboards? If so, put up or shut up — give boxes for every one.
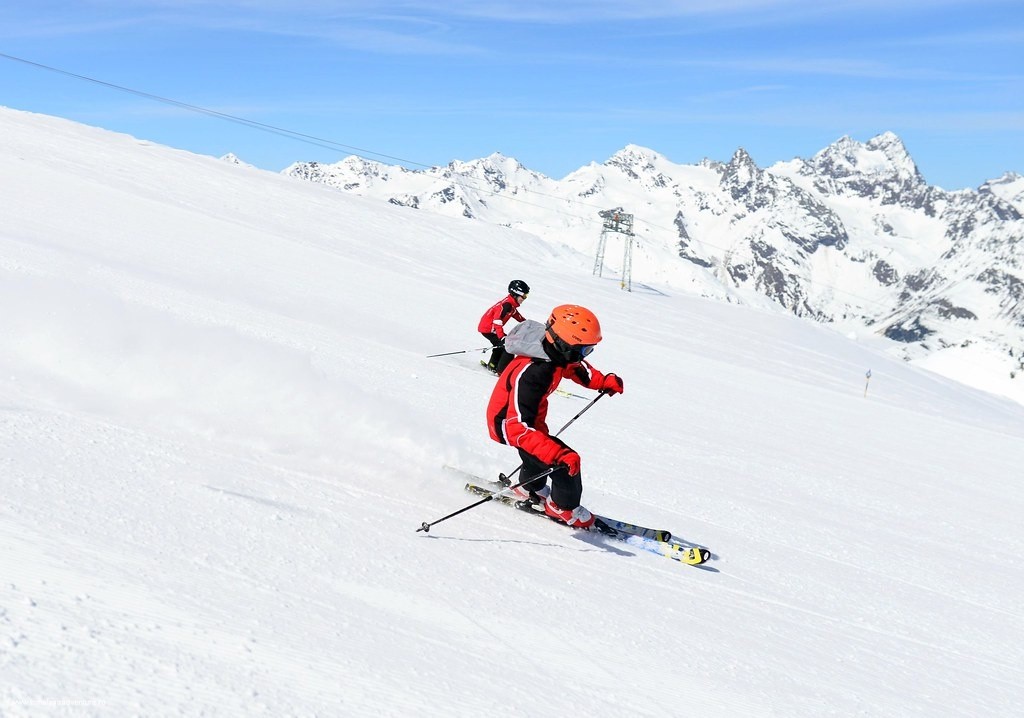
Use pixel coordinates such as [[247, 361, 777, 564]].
[[479, 359, 594, 404], [443, 465, 710, 565]]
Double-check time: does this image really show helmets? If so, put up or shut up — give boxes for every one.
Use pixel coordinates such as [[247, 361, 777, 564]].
[[508, 280, 531, 300], [546, 303, 602, 362]]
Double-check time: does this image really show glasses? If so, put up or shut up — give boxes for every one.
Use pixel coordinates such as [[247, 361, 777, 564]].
[[520, 295, 527, 299], [576, 346, 594, 356]]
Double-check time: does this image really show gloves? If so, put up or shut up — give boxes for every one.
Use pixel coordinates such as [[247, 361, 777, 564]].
[[555, 449, 581, 475], [602, 373, 623, 396]]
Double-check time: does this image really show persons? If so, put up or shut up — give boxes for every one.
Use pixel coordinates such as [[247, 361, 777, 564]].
[[485, 305, 624, 528], [478, 280, 530, 377]]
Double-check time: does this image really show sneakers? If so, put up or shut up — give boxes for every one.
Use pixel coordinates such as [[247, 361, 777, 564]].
[[513, 478, 550, 501], [488, 360, 498, 373], [544, 493, 593, 527]]
